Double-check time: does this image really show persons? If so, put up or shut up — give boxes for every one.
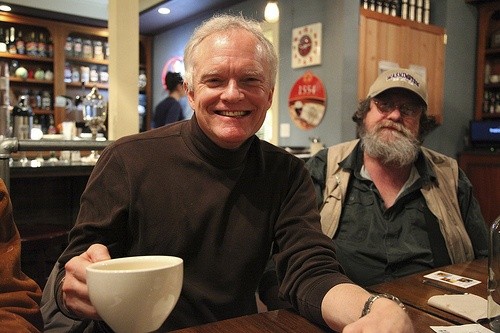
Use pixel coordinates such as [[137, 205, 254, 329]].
[[139, 71, 186, 134], [0, 178, 44, 333], [39, 16, 414, 333], [259, 66, 490, 313]]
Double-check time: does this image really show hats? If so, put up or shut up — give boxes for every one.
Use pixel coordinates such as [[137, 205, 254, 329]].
[[368, 69, 430, 111]]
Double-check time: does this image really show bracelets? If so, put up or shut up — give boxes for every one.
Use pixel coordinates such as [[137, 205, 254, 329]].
[[359, 292, 407, 320]]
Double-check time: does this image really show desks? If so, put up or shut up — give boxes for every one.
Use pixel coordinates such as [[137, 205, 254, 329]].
[[366, 257, 491, 329], [172, 290, 459, 333]]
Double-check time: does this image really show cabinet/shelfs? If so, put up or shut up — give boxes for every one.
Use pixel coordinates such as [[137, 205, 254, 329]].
[[0, 11, 154, 158], [468, 0, 500, 121]]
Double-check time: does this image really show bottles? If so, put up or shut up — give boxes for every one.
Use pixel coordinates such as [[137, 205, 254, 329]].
[[0, 23, 109, 139], [482, 64, 499, 115], [309, 137, 324, 158]]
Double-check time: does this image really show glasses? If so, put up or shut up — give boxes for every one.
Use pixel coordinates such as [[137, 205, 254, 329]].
[[372, 98, 419, 116]]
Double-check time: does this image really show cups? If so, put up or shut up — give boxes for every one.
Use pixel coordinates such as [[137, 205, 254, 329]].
[[85, 256, 184, 333]]
